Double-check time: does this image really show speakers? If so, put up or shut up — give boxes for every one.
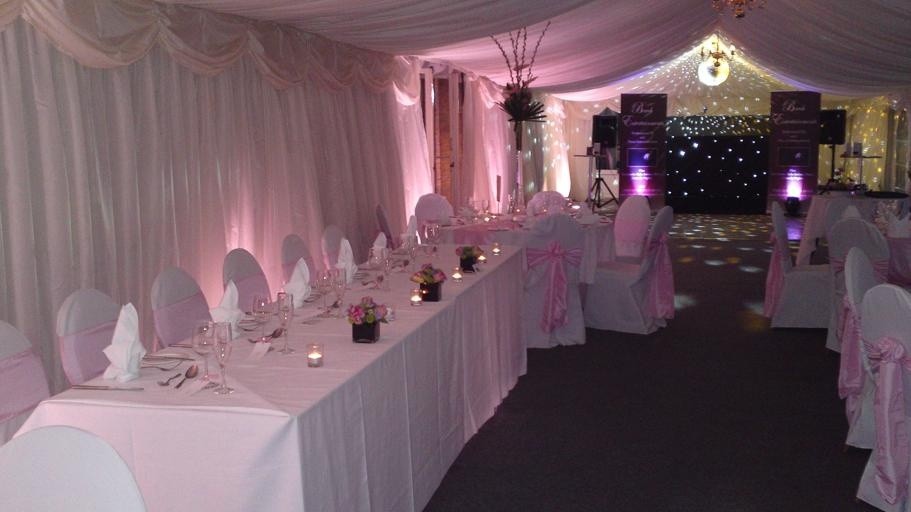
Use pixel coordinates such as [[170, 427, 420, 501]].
[[819, 109, 846, 145], [591, 114, 618, 149]]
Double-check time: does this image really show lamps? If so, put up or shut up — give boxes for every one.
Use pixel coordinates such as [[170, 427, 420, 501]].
[[710, 0, 769, 20], [698, 30, 736, 68]]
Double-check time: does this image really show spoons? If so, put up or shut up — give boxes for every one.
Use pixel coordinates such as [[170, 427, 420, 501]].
[[157, 373, 181, 386], [176, 365, 199, 388]]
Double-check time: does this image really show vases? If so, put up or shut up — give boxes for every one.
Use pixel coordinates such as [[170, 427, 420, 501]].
[[351, 323, 381, 344], [458, 256, 475, 274], [418, 283, 440, 303], [516, 123, 523, 212]]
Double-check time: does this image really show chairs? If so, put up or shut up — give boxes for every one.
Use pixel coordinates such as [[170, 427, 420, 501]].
[[772, 201, 835, 329], [585, 207, 673, 335], [0, 319, 49, 445], [831, 218, 888, 287], [320, 225, 345, 271], [281, 234, 316, 286], [1, 427, 148, 512], [843, 285, 911, 460], [376, 204, 404, 250], [151, 267, 213, 348], [613, 196, 649, 265], [56, 289, 123, 387], [527, 191, 566, 216], [415, 193, 453, 244], [844, 246, 877, 339], [223, 248, 272, 317], [524, 213, 587, 348]]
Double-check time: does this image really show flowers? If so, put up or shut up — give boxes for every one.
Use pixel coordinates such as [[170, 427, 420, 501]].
[[456, 246, 483, 258], [489, 22, 549, 123], [410, 264, 446, 284], [346, 296, 387, 325]]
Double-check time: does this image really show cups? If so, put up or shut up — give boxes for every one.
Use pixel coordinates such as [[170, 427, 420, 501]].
[[453, 267, 463, 283], [277, 294, 295, 354], [382, 248, 393, 291], [385, 304, 395, 321], [307, 343, 324, 368], [254, 297, 272, 342], [410, 289, 422, 305], [212, 323, 233, 389], [482, 199, 489, 214], [333, 269, 347, 318], [317, 271, 332, 318], [192, 321, 214, 383]]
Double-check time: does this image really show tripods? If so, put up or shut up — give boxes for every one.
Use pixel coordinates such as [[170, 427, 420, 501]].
[[584, 148, 619, 215]]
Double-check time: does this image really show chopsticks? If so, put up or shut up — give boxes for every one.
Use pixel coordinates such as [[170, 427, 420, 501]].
[[72, 384, 144, 392]]
